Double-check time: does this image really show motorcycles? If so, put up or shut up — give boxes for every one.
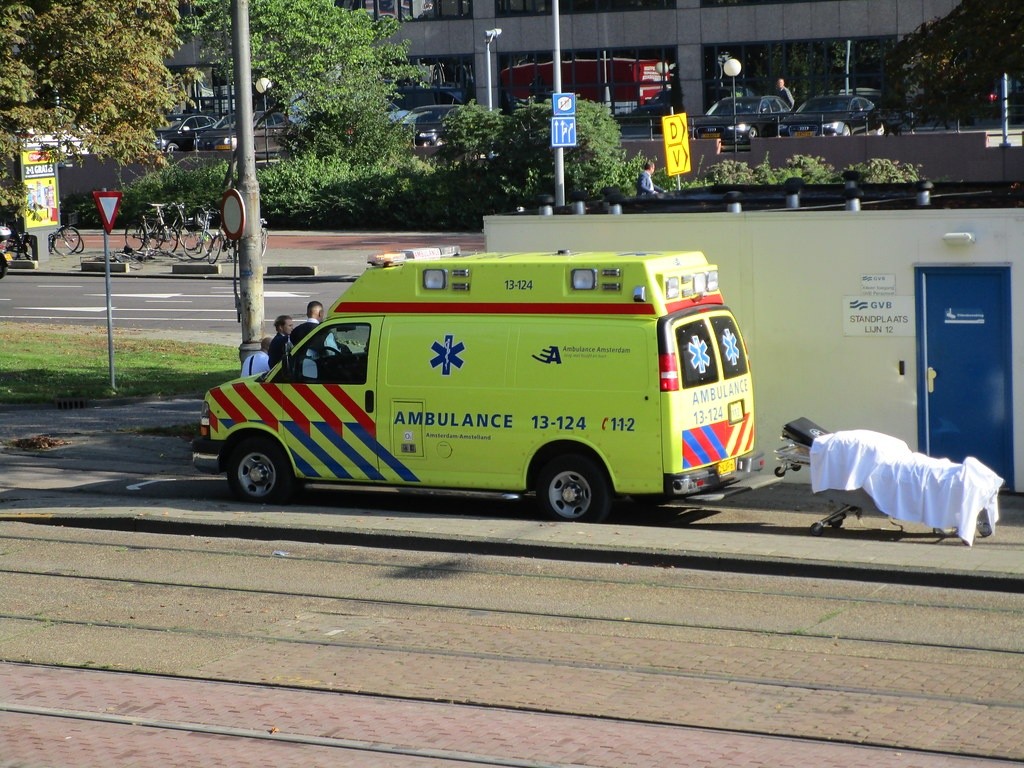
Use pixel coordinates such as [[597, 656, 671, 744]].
[[0, 227, 13, 278]]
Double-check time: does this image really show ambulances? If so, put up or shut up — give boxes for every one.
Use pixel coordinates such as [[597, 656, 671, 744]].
[[191, 245, 767, 521]]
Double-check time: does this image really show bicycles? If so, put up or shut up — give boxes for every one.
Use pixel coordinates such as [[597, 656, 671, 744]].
[[123, 202, 269, 263], [2, 207, 85, 261]]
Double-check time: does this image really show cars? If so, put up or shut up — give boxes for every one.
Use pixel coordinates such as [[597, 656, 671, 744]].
[[154, 114, 220, 152], [195, 111, 297, 150], [390, 105, 461, 146], [775, 95, 889, 137], [689, 96, 792, 142]]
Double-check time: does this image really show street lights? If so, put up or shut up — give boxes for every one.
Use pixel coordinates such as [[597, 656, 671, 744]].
[[255, 77, 274, 166], [484, 28, 503, 112], [723, 57, 742, 152]]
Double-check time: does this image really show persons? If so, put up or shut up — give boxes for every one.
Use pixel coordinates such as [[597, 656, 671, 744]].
[[242, 337, 272, 378], [291, 301, 339, 359], [766, 79, 794, 109], [268, 315, 293, 369], [30, 180, 54, 210], [636, 162, 666, 196]]
